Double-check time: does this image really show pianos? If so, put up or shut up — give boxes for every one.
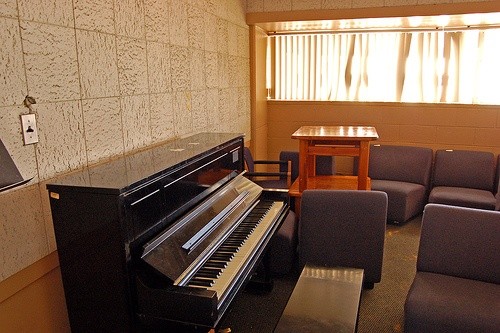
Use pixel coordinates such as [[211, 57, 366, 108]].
[[46, 133, 290, 333]]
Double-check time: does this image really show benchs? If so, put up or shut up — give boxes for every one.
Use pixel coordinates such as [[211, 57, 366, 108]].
[[274, 260, 366, 333]]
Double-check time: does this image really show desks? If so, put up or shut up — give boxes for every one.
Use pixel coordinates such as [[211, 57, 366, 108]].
[[288, 125, 379, 218]]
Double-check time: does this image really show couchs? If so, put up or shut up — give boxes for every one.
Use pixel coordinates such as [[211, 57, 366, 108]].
[[280, 145, 500, 333]]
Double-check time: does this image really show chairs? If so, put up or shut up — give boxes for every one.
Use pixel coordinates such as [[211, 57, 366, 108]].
[[243, 147, 287, 188]]
